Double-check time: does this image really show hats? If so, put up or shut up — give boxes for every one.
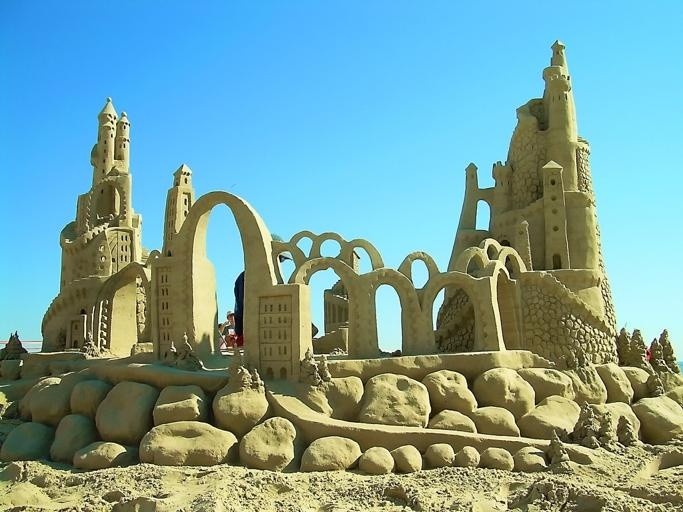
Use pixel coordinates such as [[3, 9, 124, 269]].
[[227, 311, 234, 319]]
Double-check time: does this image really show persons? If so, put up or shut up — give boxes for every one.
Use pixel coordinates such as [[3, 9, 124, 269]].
[[218, 310, 243, 348], [646, 346, 652, 361]]
